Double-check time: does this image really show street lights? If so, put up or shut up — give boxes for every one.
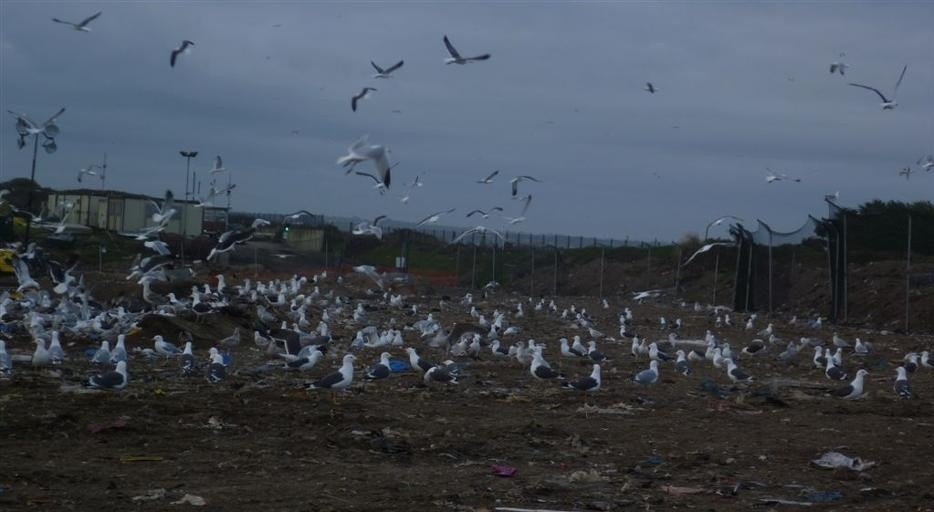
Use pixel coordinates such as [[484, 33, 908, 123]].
[[178, 150, 198, 240]]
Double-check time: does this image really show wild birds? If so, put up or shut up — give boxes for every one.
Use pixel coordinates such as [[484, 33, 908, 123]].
[[281, 210, 314, 226], [207, 210, 271, 261], [644, 82, 659, 93], [6, 107, 67, 138], [765, 166, 801, 185], [77, 164, 100, 183], [0, 241, 617, 406], [351, 88, 376, 112], [170, 40, 195, 67], [371, 60, 404, 78], [618, 289, 934, 409], [830, 62, 850, 75], [681, 215, 744, 267], [848, 64, 907, 110], [116, 190, 182, 240], [899, 153, 934, 180], [50, 10, 102, 32], [443, 35, 491, 65], [336, 134, 543, 246], [186, 154, 237, 208], [1, 189, 94, 236]]
